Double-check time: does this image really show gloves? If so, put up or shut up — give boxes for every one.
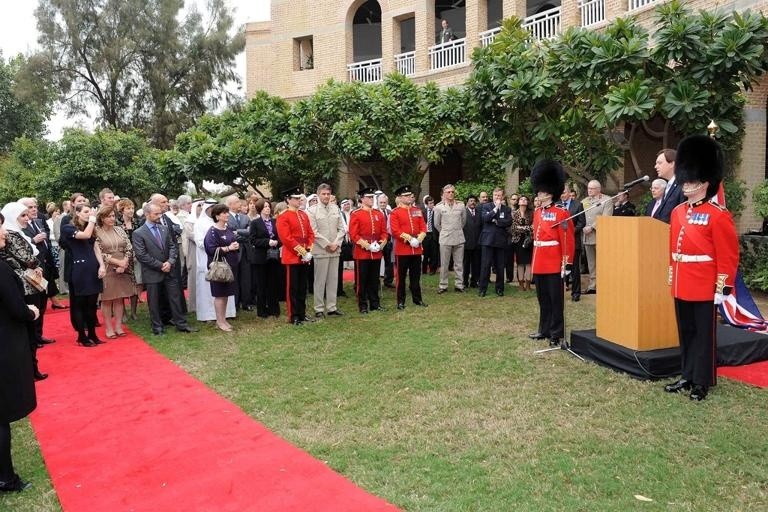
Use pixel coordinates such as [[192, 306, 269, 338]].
[[301, 252, 313, 262], [369, 240, 380, 253], [409, 237, 420, 248]]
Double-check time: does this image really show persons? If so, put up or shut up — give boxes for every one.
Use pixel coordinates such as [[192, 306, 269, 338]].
[[1, 196, 67, 487], [439, 19, 454, 43], [525, 181, 575, 346], [643, 179, 665, 217], [664, 179, 740, 401], [57, 183, 534, 348], [556, 180, 637, 303], [655, 148, 687, 223]]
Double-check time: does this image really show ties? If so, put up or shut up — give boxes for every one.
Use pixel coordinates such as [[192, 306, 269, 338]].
[[163, 215, 166, 226], [153, 225, 163, 251], [428, 209, 433, 231], [383, 210, 386, 221], [235, 214, 240, 228]]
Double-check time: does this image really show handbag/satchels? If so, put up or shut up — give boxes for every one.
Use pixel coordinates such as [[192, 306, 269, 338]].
[[23, 268, 49, 293], [266, 248, 280, 262], [206, 262, 235, 284]]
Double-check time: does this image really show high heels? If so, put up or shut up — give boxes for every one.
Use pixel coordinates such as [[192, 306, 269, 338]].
[[77, 334, 105, 346], [51, 303, 69, 310], [215, 320, 234, 332]]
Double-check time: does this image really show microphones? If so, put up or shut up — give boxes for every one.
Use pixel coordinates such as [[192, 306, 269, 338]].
[[624, 175, 649, 190]]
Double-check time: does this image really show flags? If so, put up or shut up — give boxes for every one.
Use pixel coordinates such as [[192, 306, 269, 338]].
[[709, 179, 768, 335]]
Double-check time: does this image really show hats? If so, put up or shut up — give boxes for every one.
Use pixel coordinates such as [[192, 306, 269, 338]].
[[281, 186, 303, 198], [357, 187, 376, 197], [395, 185, 413, 195]]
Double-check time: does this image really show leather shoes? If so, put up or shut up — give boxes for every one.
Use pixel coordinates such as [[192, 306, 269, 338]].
[[360, 306, 388, 314], [179, 326, 200, 333], [415, 301, 428, 307], [39, 337, 55, 343], [397, 302, 404, 309], [292, 310, 345, 326], [34, 368, 48, 379], [0, 475, 33, 492], [105, 327, 127, 339]]
